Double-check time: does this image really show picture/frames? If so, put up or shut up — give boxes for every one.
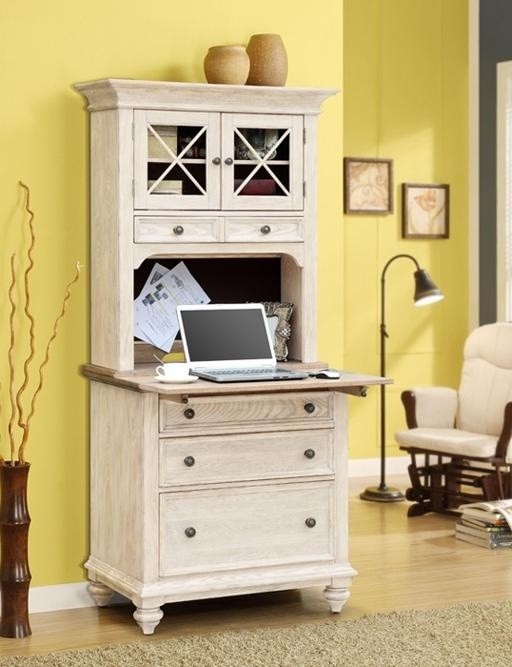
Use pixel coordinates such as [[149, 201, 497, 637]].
[[342, 155, 394, 216], [400, 182, 449, 238]]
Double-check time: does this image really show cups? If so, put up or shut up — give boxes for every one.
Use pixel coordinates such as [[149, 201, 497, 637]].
[[155, 362, 190, 376]]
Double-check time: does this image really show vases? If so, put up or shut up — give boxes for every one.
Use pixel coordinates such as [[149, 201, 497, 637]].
[[1, 458, 32, 638]]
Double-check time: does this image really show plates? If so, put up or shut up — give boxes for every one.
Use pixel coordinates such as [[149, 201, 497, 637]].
[[153, 375, 200, 384]]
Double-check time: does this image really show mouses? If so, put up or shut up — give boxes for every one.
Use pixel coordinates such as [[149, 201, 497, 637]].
[[315, 367, 341, 378]]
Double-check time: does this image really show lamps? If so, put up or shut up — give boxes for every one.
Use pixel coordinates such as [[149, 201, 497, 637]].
[[360, 253, 446, 502]]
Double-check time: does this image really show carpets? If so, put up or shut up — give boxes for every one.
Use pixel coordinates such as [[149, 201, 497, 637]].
[[2, 599, 510, 667]]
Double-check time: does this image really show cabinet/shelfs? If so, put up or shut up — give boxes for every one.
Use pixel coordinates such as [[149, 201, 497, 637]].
[[76, 75, 388, 638]]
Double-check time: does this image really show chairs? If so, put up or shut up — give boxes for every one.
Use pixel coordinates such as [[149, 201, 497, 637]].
[[400, 324, 512, 525]]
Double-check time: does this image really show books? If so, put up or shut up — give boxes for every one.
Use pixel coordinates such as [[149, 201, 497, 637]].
[[446, 499, 512, 550]]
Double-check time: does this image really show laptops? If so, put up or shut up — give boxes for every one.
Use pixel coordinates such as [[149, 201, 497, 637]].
[[176, 302, 309, 383]]
